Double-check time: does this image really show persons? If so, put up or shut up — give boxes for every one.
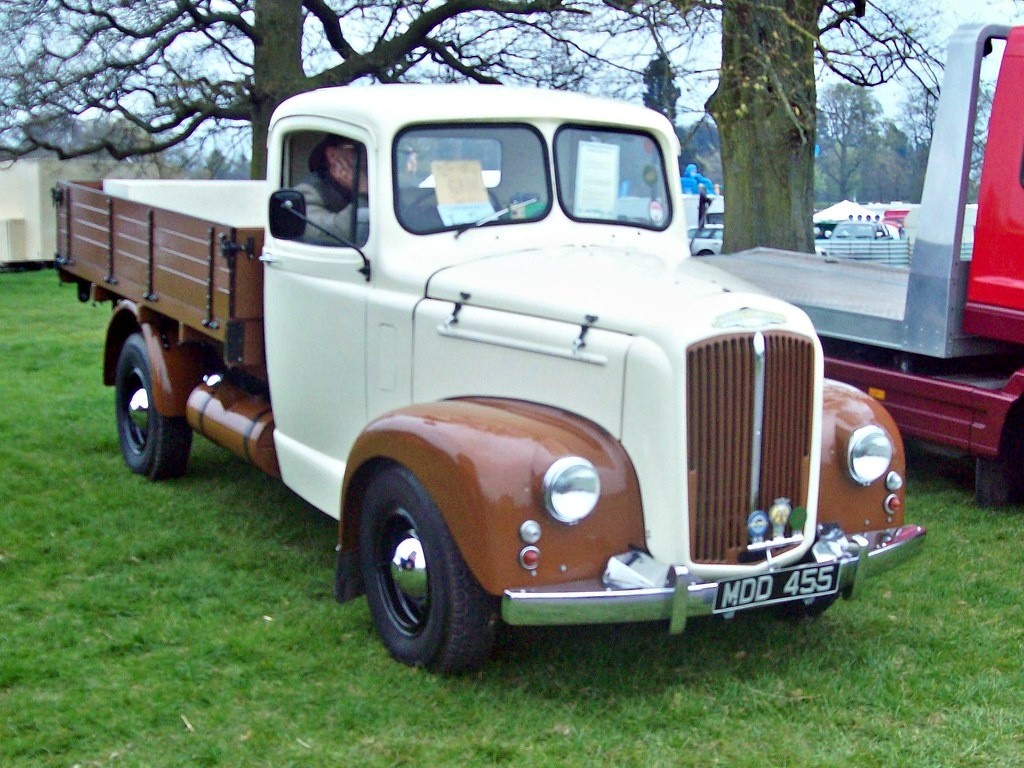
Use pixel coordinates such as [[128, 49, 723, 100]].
[[283, 134, 421, 242]]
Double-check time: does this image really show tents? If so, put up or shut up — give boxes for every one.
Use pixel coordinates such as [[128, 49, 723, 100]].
[[813, 199, 882, 226]]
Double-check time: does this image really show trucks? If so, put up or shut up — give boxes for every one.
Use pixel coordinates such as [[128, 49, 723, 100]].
[[49, 81, 930, 680], [686, 21, 1024, 506]]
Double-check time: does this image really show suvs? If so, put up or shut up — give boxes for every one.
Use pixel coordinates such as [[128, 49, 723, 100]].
[[691, 223, 725, 259]]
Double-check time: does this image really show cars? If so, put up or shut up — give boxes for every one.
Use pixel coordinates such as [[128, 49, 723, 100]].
[[825, 220, 896, 240]]
